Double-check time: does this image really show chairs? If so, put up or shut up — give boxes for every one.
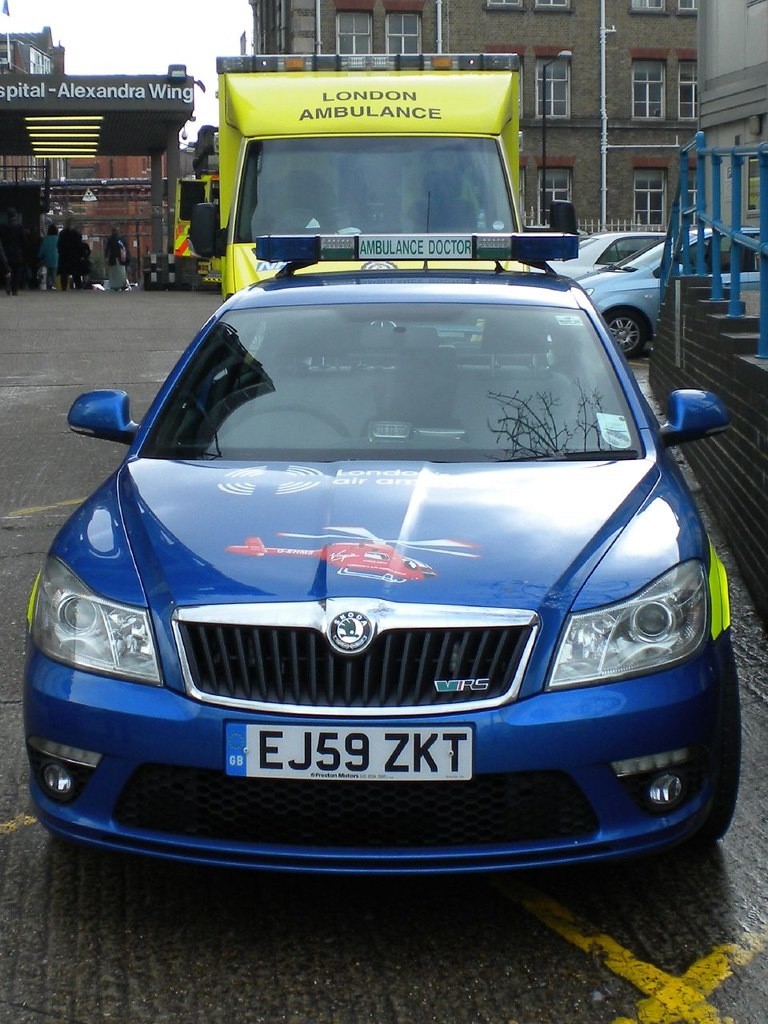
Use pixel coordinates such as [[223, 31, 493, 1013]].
[[268, 307, 402, 437], [449, 310, 574, 450], [401, 162, 479, 231], [401, 322, 455, 378]]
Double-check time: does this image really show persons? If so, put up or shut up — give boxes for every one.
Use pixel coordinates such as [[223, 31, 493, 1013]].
[[103, 227, 129, 290], [0, 207, 37, 296], [39, 224, 59, 290], [56, 219, 84, 290]]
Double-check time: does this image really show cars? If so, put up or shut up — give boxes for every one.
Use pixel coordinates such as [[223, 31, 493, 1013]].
[[570, 224, 760, 358], [529, 230, 669, 280], [20, 231, 743, 883]]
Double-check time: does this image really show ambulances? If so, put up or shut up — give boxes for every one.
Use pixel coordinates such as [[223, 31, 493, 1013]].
[[172, 168, 221, 291], [189, 51, 581, 300]]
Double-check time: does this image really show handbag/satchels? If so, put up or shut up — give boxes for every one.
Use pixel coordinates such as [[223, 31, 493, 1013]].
[[120, 246, 126, 263]]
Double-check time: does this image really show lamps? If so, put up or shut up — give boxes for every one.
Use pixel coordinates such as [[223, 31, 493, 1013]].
[[190, 115, 196, 121], [194, 79, 207, 93], [168, 64, 187, 79]]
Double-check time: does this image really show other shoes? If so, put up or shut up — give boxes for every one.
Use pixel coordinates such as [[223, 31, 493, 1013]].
[[5, 288, 16, 296]]
